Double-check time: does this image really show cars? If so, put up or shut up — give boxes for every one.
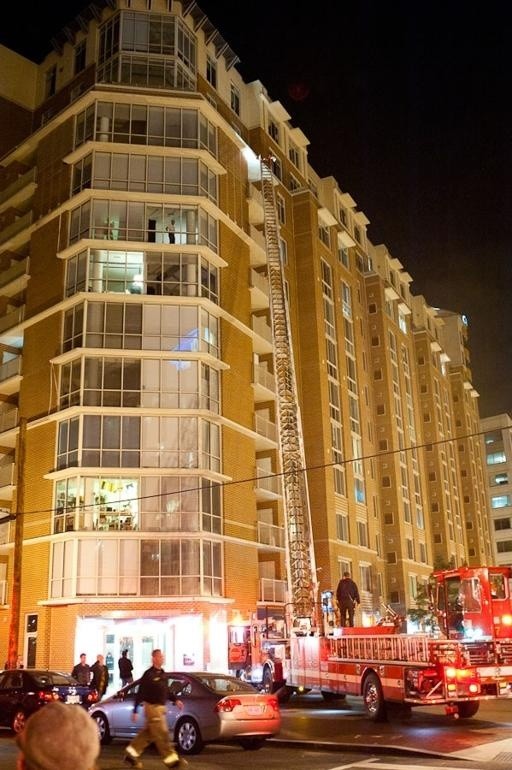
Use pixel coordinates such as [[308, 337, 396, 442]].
[[85, 672, 281, 755], [0, 669, 99, 734]]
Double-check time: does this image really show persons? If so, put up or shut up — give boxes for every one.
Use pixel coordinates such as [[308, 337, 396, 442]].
[[16, 701, 102, 769], [4, 655, 24, 669], [71, 653, 91, 686], [90, 655, 109, 697], [105, 216, 114, 240], [336, 571, 360, 626], [123, 649, 189, 770], [118, 649, 135, 694], [165, 220, 175, 244]]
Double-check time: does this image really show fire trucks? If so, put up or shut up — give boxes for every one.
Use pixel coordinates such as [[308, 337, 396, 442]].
[[226, 604, 512, 721], [427, 566, 512, 639]]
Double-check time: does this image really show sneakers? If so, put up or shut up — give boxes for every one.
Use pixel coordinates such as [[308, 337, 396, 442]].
[[169, 759, 189, 769], [123, 755, 143, 769]]
